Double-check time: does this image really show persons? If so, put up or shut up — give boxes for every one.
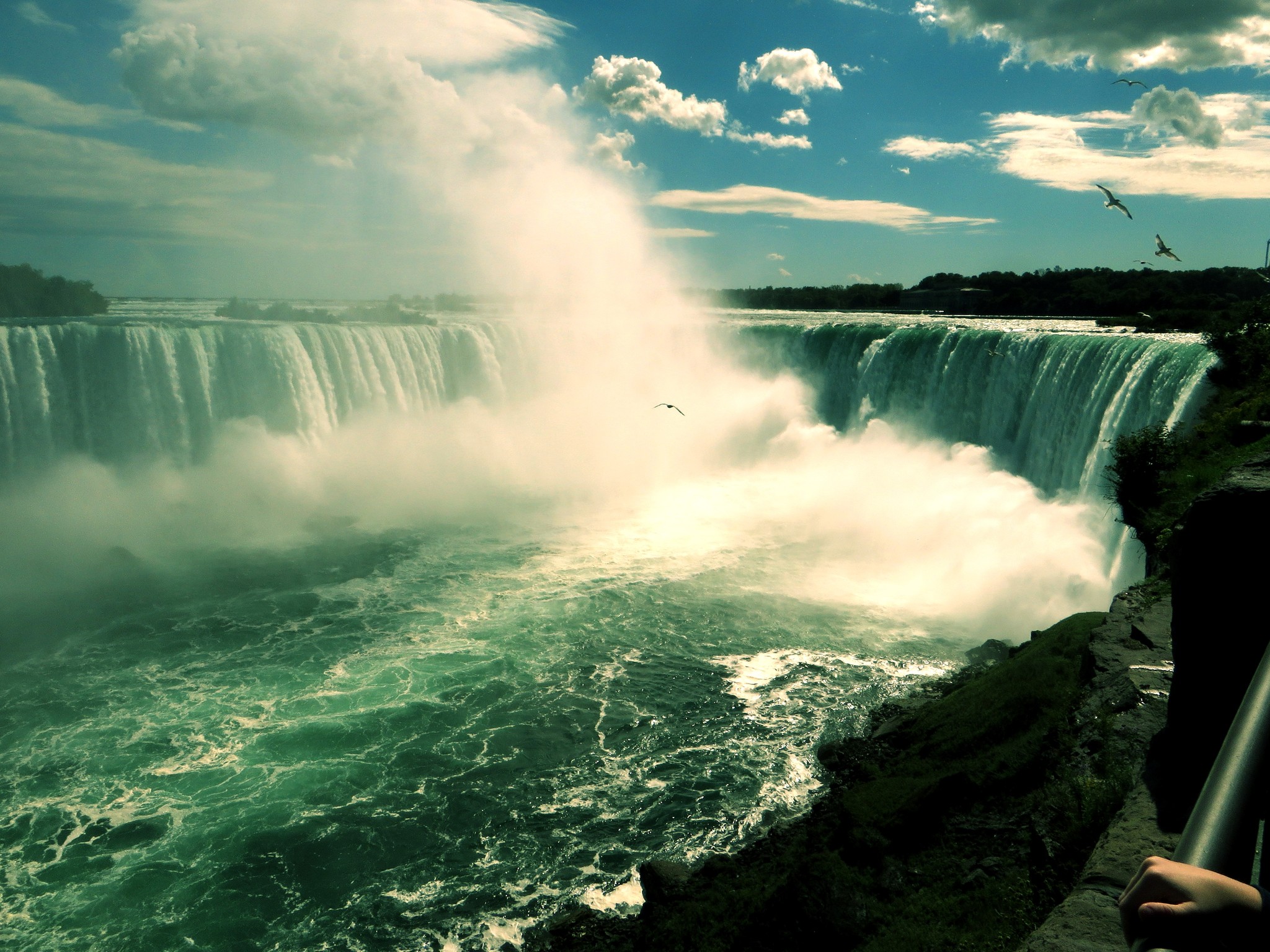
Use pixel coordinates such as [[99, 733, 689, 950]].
[[1118, 856, 1270, 952]]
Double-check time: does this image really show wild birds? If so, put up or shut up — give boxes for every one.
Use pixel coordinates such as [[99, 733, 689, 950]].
[[983, 348, 1005, 356], [1133, 260, 1154, 266], [653, 404, 685, 416], [1154, 234, 1181, 261], [1096, 184, 1133, 221], [1137, 312, 1153, 320]]
